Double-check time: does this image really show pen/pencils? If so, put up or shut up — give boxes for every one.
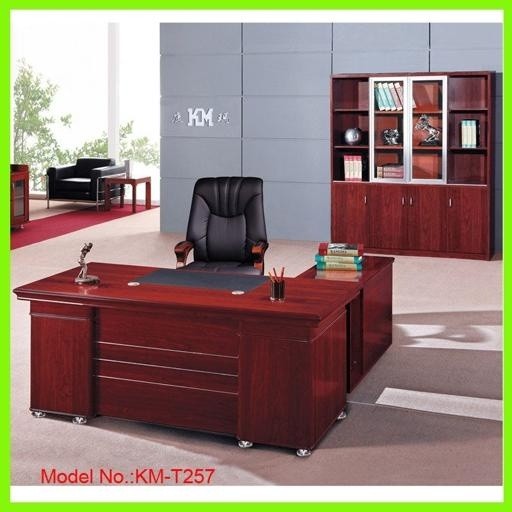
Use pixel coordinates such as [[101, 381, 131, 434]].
[[268, 267, 285, 283]]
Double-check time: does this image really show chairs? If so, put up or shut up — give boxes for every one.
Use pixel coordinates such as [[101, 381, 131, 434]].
[[46, 157, 126, 210], [173, 175, 268, 276]]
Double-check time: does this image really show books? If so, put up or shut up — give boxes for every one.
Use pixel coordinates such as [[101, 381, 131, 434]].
[[314, 253, 366, 264], [317, 263, 363, 271], [461, 120, 467, 148], [472, 119, 482, 148], [318, 240, 365, 257], [373, 82, 385, 112], [401, 85, 418, 109], [343, 153, 404, 182], [382, 82, 397, 112], [388, 82, 403, 111], [377, 82, 391, 112], [467, 120, 472, 148]]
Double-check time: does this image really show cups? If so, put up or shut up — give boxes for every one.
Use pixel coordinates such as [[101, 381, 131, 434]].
[[269, 279, 285, 302]]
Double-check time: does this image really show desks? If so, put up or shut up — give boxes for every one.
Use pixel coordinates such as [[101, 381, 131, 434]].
[[103, 175, 152, 213]]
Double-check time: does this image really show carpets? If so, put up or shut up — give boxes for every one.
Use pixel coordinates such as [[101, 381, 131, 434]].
[[10, 204, 160, 250]]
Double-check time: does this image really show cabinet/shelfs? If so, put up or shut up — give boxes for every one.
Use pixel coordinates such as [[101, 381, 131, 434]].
[[329, 71, 496, 261], [10, 164, 29, 229]]
